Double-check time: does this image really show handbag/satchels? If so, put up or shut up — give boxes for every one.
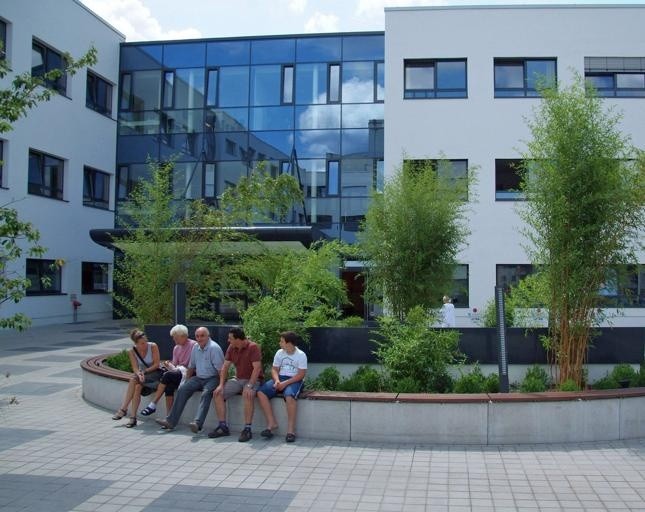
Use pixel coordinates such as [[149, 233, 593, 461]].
[[140, 367, 169, 397]]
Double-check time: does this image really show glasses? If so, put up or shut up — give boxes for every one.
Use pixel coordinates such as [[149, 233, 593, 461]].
[[135, 333, 145, 344]]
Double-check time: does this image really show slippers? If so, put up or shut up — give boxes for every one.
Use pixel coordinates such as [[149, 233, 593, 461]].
[[285, 433, 295, 442], [261, 429, 273, 438]]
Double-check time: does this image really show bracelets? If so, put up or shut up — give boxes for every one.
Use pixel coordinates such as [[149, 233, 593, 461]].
[[246, 382, 255, 388]]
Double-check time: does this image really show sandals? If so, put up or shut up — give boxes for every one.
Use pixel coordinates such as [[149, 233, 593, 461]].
[[140, 406, 156, 416], [125, 417, 137, 428], [111, 408, 128, 420]]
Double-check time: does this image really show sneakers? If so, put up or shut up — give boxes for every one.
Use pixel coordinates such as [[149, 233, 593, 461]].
[[189, 422, 199, 433], [208, 423, 231, 438], [155, 417, 174, 428], [238, 429, 252, 442]]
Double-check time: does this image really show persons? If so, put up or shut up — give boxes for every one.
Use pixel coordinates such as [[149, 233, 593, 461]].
[[141, 324, 197, 429], [156, 326, 225, 433], [256, 331, 308, 443], [440, 295, 455, 328], [111, 330, 162, 429], [208, 328, 265, 441]]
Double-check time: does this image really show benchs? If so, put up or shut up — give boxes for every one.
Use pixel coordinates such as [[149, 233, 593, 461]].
[[80, 354, 133, 412]]
